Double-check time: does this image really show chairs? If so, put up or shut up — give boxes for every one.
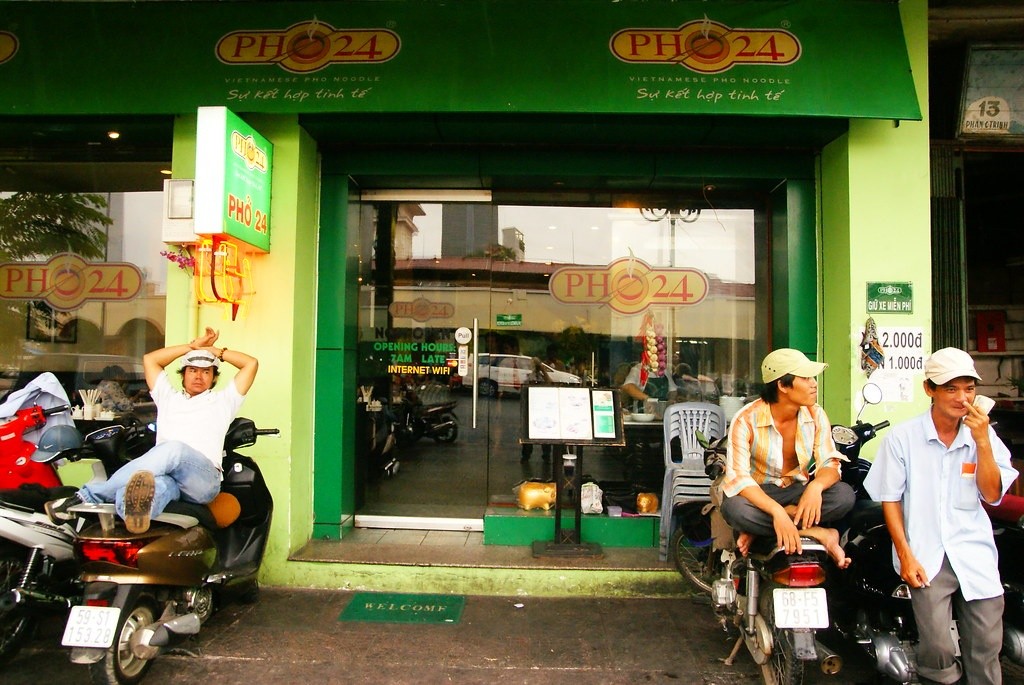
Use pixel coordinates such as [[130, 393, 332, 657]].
[[658, 402, 727, 563]]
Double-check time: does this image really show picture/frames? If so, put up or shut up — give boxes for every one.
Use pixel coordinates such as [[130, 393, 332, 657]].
[[26, 301, 78, 344]]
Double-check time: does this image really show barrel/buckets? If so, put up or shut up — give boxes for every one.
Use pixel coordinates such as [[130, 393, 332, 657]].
[[719, 396, 744, 422]]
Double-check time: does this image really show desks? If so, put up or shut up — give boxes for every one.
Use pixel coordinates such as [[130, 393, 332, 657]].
[[73, 410, 133, 436], [355, 407, 397, 496], [625, 420, 663, 443]]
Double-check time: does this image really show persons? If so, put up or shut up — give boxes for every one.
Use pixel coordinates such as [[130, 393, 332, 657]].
[[470, 332, 532, 466], [92, 365, 139, 411], [623, 355, 679, 421], [673, 362, 746, 405], [721, 349, 856, 569], [863, 347, 1019, 685], [517, 356, 556, 464], [45, 327, 260, 534]]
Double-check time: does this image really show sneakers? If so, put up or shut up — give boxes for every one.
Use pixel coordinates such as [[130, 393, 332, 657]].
[[43, 492, 85, 524], [125, 470, 155, 533]]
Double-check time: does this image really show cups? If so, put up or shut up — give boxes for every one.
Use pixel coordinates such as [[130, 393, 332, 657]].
[[84, 405, 115, 419]]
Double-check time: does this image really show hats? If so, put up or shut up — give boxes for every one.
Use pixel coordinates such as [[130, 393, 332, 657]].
[[761, 348, 828, 383], [30, 424, 83, 461], [179, 348, 221, 369], [925, 345, 984, 384]]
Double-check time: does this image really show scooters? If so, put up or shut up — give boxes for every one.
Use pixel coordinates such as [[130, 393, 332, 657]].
[[0, 402, 281, 685], [357, 372, 463, 482], [666, 382, 1024, 684]]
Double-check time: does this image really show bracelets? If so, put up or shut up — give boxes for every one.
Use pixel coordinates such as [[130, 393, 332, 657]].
[[218, 347, 228, 364]]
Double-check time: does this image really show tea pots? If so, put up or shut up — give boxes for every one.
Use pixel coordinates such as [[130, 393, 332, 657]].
[[71, 405, 84, 417]]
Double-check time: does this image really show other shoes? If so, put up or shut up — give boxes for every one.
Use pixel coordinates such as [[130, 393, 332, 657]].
[[543, 458, 552, 464], [520, 455, 531, 463]]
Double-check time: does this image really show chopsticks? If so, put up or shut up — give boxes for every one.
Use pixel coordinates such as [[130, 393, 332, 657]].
[[78, 389, 102, 405]]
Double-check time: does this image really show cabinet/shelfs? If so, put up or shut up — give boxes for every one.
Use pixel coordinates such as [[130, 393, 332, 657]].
[[969, 350, 1024, 402]]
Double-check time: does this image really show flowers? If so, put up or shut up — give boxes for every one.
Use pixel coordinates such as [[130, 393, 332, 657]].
[[160, 247, 198, 275]]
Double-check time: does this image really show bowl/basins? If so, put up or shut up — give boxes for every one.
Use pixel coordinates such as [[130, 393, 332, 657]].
[[644, 401, 673, 420], [631, 413, 655, 422]]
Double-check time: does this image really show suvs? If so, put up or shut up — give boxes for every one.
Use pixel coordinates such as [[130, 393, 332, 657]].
[[463, 352, 583, 398]]
